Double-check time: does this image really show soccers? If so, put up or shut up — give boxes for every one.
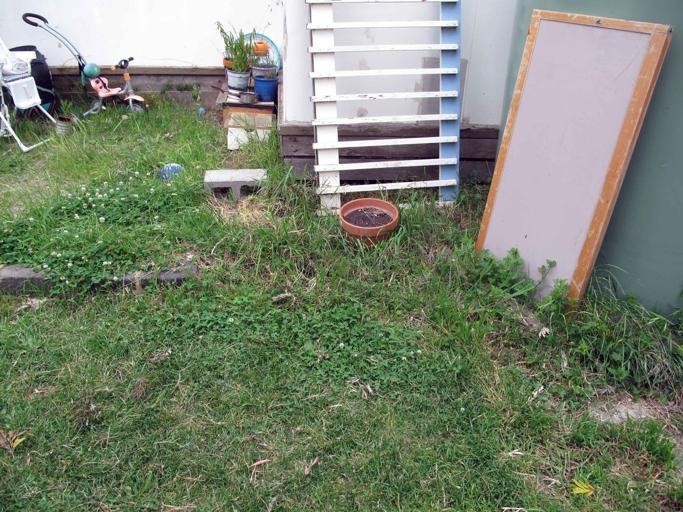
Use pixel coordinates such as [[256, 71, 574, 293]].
[[159, 163, 182, 181]]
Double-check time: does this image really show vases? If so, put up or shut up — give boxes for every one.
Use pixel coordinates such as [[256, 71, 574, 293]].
[[337, 198, 400, 246]]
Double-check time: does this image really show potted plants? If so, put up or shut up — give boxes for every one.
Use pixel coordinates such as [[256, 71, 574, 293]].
[[213, 20, 279, 102]]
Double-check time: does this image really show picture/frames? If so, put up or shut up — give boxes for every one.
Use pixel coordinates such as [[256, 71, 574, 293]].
[[473, 7, 673, 322]]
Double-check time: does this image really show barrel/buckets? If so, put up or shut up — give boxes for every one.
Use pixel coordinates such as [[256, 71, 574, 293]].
[[4, 46, 57, 119], [227, 70, 251, 100], [255, 75, 279, 102]]
